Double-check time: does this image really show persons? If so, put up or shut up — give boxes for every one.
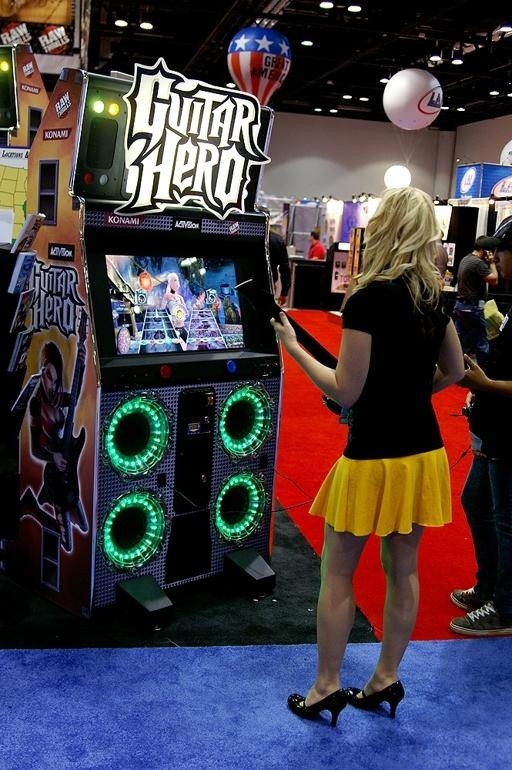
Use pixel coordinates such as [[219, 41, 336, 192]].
[[449, 215, 512, 637], [270, 187, 464, 726], [434, 231, 448, 276], [305, 232, 325, 261], [258, 206, 291, 306], [453, 234, 499, 387]]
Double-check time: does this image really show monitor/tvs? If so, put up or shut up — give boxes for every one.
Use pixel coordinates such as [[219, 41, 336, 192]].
[[105, 255, 246, 355]]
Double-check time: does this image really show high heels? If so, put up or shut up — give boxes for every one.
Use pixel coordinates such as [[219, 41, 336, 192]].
[[346, 679, 405, 718], [287, 687, 348, 727]]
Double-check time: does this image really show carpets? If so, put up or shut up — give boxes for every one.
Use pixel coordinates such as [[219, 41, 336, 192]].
[[269, 298, 510, 642], [0, 636, 512, 769]]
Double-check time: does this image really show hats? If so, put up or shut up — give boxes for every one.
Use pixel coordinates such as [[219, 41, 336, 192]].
[[474, 214, 512, 251]]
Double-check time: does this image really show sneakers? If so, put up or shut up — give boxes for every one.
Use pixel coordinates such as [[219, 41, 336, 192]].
[[449, 600, 512, 636], [450, 585, 490, 610]]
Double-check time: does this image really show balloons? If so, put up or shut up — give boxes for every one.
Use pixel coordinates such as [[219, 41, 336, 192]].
[[385, 166, 411, 192], [227, 27, 292, 107], [383, 68, 445, 131]]
